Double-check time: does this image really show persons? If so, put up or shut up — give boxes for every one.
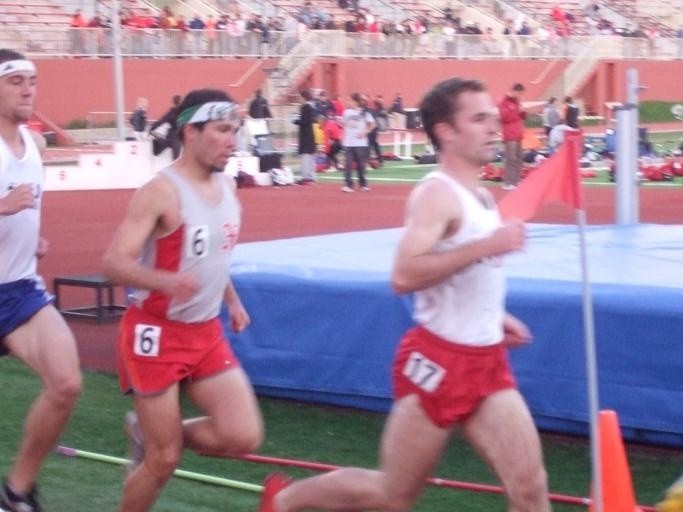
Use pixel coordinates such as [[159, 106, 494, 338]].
[[260, 76, 551, 512], [128, 78, 585, 190], [0, 48, 83, 511], [70, 0, 683, 58], [102, 89, 266, 512]]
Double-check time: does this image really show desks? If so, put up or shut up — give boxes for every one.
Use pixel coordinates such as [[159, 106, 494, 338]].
[[53, 274, 127, 324]]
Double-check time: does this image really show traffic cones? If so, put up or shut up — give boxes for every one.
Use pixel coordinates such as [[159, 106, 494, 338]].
[[586, 409, 643, 512]]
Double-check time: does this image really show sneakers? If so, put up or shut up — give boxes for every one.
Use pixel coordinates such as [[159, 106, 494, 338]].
[[120, 410, 147, 482], [257, 473, 293, 511], [1, 481, 45, 511], [341, 185, 372, 194]]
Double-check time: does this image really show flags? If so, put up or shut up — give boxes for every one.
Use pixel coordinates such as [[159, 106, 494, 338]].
[[495, 141, 580, 221]]
